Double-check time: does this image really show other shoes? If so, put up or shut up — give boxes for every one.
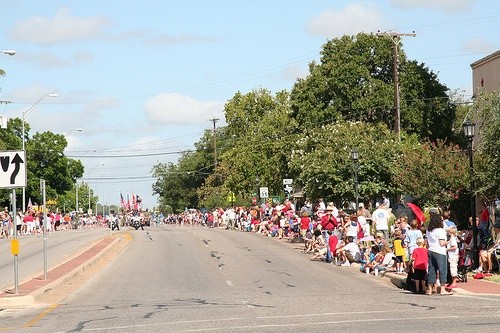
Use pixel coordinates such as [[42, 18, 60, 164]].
[[424, 290, 432, 295], [446, 284, 458, 289], [340, 261, 351, 267], [439, 290, 453, 295]]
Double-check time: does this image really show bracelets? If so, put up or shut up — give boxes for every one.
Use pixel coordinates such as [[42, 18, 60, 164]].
[[450, 240, 451, 243]]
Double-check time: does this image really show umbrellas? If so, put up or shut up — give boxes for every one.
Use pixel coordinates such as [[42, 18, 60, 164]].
[[406, 202, 426, 222]]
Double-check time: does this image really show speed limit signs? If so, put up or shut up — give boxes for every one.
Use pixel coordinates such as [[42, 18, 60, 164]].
[[259, 186, 269, 198]]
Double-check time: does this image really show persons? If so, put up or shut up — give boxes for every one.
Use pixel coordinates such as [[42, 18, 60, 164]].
[[409, 237, 429, 294], [394, 230, 407, 272], [464, 227, 480, 267], [445, 225, 458, 289], [405, 219, 423, 273], [131, 212, 144, 230], [159, 212, 164, 225], [110, 214, 120, 230], [425, 213, 453, 295], [0, 207, 110, 239], [441, 212, 456, 227], [472, 223, 500, 274], [152, 213, 157, 225], [165, 198, 410, 273], [480, 201, 489, 236]]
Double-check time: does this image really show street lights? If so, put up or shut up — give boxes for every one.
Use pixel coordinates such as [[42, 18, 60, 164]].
[[460, 121, 479, 270], [89, 173, 111, 215], [76, 162, 105, 211], [22, 93, 58, 213], [351, 150, 360, 211]]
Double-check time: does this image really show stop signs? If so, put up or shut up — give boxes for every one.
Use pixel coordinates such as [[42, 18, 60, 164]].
[[253, 197, 256, 205]]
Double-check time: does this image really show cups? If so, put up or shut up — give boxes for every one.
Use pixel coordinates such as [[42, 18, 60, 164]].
[[365, 267, 369, 275], [375, 269, 378, 276]]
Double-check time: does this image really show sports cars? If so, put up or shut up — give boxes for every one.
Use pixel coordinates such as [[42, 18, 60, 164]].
[[128, 213, 142, 230]]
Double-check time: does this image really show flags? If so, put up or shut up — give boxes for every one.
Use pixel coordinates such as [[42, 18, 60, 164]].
[[136, 195, 142, 211], [127, 194, 130, 209], [120, 193, 126, 208], [132, 195, 136, 210]]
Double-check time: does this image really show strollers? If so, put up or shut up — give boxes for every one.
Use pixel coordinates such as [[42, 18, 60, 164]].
[[456, 230, 472, 283]]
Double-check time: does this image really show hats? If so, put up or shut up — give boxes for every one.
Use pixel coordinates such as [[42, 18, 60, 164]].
[[324, 206, 334, 211]]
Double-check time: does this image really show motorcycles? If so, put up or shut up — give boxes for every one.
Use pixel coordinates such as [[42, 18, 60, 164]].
[[107, 214, 118, 230]]
